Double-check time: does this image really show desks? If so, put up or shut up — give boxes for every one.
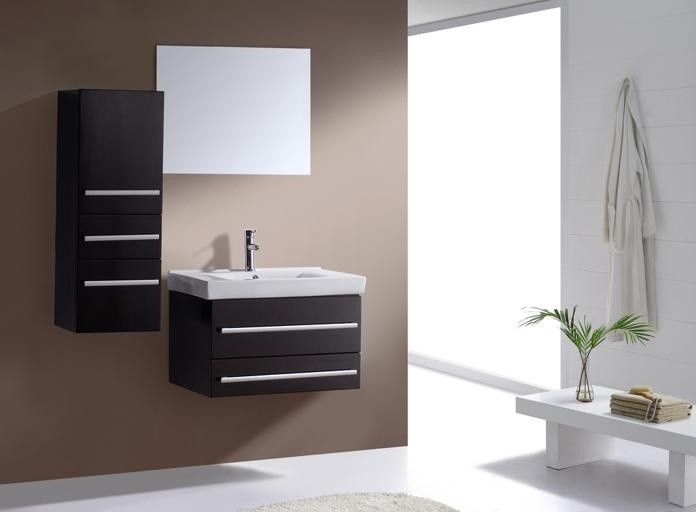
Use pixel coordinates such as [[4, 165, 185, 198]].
[[517, 383, 695, 508]]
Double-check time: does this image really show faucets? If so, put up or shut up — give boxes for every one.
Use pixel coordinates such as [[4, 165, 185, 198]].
[[246, 229, 259, 271]]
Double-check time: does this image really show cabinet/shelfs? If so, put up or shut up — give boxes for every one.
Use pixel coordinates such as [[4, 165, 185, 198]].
[[53, 88, 164, 333], [168, 289, 361, 398]]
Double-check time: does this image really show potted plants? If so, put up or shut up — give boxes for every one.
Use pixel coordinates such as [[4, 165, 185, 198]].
[[518, 303, 657, 401]]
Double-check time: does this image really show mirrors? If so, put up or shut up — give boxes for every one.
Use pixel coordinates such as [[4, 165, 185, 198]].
[[156, 45, 310, 177]]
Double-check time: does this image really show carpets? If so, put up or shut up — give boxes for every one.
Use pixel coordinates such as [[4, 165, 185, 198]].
[[238, 491, 459, 512]]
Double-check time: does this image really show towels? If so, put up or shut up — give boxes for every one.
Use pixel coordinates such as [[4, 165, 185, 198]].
[[609, 393, 692, 422]]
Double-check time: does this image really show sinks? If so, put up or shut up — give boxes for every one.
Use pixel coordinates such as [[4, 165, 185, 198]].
[[166, 267, 367, 301]]
